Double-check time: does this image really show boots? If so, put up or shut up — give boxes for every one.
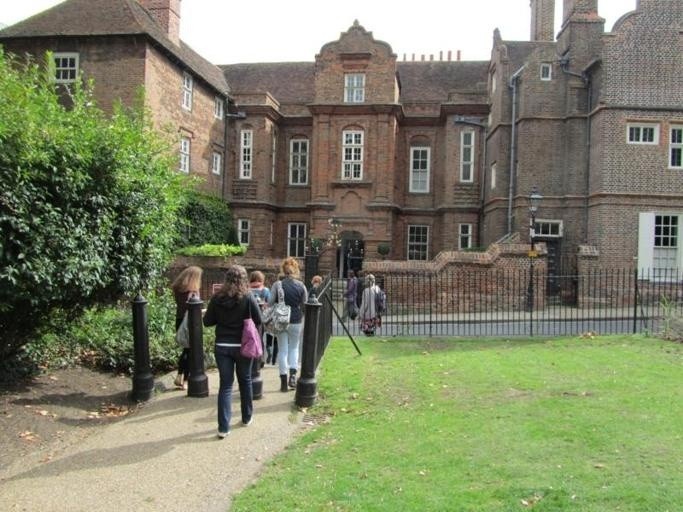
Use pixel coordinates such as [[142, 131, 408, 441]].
[[266, 346, 273, 363], [280, 374, 288, 392], [288, 368, 297, 388]]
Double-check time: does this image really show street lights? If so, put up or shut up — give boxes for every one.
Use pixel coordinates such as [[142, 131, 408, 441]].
[[522, 181, 543, 313]]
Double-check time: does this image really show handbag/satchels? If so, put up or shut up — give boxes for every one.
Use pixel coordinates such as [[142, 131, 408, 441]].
[[240, 292, 263, 359], [261, 280, 291, 335], [175, 291, 191, 348]]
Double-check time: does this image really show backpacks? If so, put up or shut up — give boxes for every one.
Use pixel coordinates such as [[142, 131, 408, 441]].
[[372, 288, 387, 314], [254, 287, 266, 312]]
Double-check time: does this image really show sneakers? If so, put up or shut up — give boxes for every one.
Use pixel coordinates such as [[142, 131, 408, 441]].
[[242, 416, 253, 426], [218, 430, 231, 438]]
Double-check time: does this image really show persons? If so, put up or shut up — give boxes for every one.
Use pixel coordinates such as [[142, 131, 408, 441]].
[[267, 256, 309, 394], [201, 264, 261, 438], [342, 267, 387, 336], [245, 270, 273, 400], [306, 275, 324, 297], [172, 265, 205, 388], [263, 273, 280, 364]]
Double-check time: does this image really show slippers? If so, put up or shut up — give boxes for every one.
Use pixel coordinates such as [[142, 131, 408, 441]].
[[174, 373, 185, 391]]
